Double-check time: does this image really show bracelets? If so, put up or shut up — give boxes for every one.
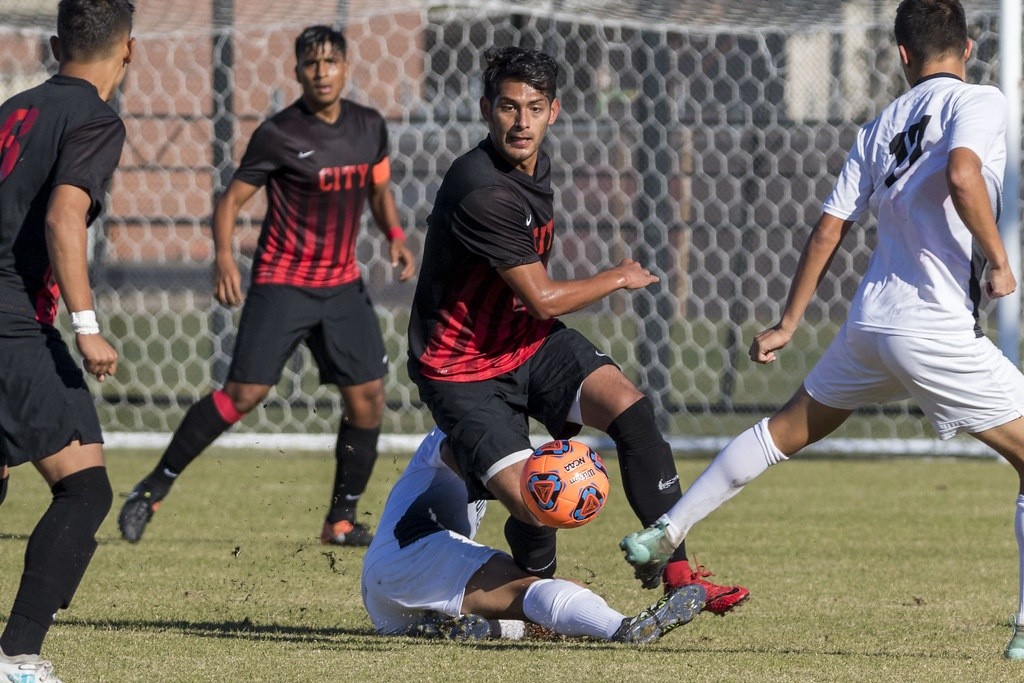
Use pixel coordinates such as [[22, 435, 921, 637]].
[[71, 310, 100, 334], [386, 226, 406, 241]]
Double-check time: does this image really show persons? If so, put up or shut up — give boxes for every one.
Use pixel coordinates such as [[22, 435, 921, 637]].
[[0, 0, 136, 683], [117, 24, 416, 547], [407, 47, 750, 617], [620, 0, 1024, 659], [362, 428, 706, 643]]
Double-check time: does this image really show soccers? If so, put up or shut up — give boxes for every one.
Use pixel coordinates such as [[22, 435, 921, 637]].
[[517, 435, 614, 533]]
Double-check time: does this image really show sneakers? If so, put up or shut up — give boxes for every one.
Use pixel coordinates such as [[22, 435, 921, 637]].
[[118, 486, 159, 544], [1004, 612, 1024, 661], [620, 514, 680, 589], [0, 641, 65, 683], [616, 584, 707, 644], [661, 559, 751, 617], [322, 512, 374, 548], [417, 614, 492, 641]]
